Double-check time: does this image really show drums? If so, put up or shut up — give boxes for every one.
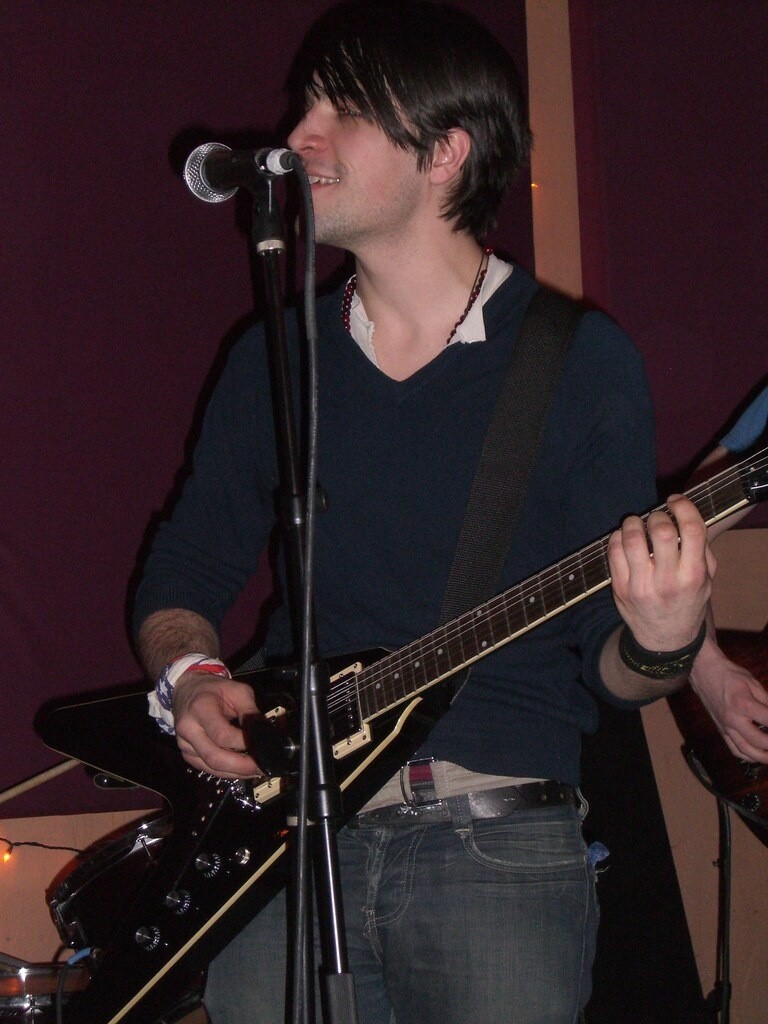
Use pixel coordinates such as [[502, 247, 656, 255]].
[[51, 805, 207, 1024]]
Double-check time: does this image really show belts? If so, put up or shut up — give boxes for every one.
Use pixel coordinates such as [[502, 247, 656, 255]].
[[346, 780, 568, 828]]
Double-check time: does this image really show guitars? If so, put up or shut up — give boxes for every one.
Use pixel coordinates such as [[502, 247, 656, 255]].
[[30, 437, 768, 1024]]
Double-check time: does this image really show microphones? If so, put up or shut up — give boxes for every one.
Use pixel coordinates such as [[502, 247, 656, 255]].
[[185, 142, 300, 203]]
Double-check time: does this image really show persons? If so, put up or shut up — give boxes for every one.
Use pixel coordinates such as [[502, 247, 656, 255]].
[[689, 391, 768, 765], [131, 0, 716, 1024]]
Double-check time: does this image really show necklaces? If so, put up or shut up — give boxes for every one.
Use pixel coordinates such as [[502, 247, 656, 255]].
[[341, 246, 494, 342]]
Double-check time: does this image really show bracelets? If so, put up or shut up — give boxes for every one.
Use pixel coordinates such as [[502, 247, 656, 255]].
[[150, 653, 232, 737], [618, 623, 708, 679]]
